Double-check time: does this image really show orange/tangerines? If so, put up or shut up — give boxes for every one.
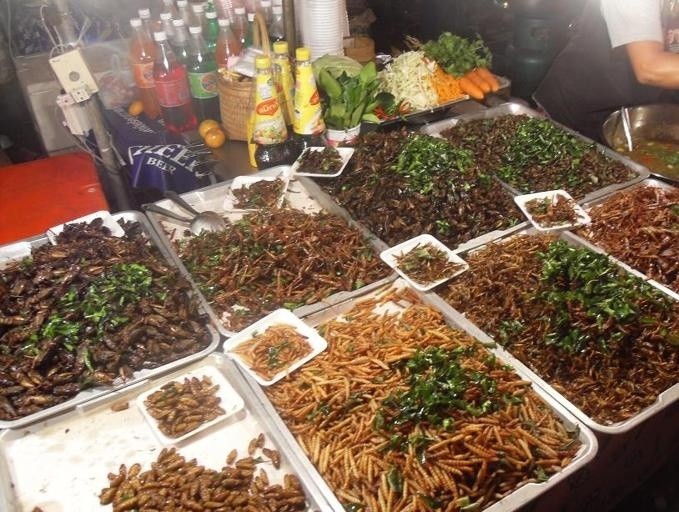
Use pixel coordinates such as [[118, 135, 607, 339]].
[[128, 99, 143, 116], [204, 128, 225, 148], [199, 119, 219, 138]]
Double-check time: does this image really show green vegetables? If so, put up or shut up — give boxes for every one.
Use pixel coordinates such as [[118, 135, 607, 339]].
[[319, 61, 380, 130]]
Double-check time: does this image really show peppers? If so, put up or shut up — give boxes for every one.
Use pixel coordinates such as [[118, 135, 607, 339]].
[[374, 98, 415, 122]]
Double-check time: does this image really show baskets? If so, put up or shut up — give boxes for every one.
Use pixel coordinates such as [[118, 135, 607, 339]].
[[219, 13, 284, 142]]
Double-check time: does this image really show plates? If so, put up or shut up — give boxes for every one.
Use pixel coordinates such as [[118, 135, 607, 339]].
[[1, 352, 335, 510], [514, 189, 592, 232], [222, 306, 328, 388], [0, 208, 221, 430], [298, 129, 536, 266], [421, 224, 678, 435], [0, 243, 32, 270], [358, 53, 512, 127], [379, 234, 471, 292], [300, 0, 344, 63], [421, 99, 651, 206], [46, 210, 126, 248], [145, 164, 402, 338], [133, 363, 248, 445], [289, 145, 357, 178], [229, 273, 601, 512], [566, 179, 679, 299], [224, 174, 291, 211]]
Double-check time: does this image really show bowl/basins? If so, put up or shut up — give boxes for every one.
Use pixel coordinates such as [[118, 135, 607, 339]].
[[599, 100, 679, 182]]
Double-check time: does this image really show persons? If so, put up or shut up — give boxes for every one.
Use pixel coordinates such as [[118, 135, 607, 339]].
[[532, 0, 679, 142]]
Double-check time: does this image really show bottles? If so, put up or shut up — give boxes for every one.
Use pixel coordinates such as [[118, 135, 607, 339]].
[[233, 6, 247, 43], [151, 28, 196, 134], [247, 59, 292, 170], [128, 17, 162, 120], [176, 0, 190, 25], [291, 45, 326, 159], [242, 12, 257, 48], [205, 8, 219, 41], [137, 6, 152, 40], [158, 11, 172, 42], [271, 40, 296, 132], [170, 19, 190, 71], [186, 25, 220, 125], [215, 17, 242, 68], [163, 0, 177, 17], [190, 1, 208, 34]]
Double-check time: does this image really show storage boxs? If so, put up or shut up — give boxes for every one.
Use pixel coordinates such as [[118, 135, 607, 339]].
[[13, 38, 134, 154]]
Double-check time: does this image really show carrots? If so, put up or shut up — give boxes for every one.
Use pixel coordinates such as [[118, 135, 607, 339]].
[[420, 32, 498, 99]]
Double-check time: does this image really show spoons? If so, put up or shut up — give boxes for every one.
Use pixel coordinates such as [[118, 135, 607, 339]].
[[162, 190, 226, 232], [139, 202, 225, 239]]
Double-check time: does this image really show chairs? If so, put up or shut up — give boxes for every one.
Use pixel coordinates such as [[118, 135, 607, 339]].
[[0, 151, 110, 246]]
